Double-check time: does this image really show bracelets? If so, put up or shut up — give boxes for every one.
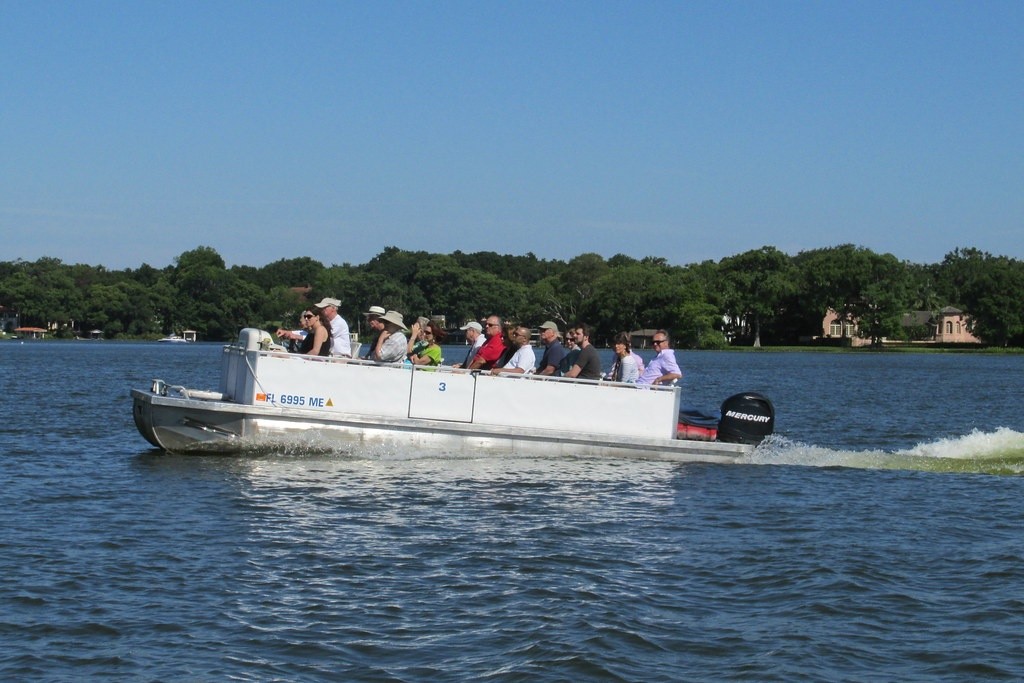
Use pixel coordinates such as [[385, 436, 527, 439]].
[[411, 336, 416, 341], [658, 376, 662, 381], [287, 333, 291, 339]]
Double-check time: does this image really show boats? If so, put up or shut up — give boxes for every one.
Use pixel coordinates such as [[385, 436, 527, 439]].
[[158, 335, 187, 342], [130, 327, 776, 464]]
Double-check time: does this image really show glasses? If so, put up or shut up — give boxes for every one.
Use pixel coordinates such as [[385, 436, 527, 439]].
[[424, 331, 431, 335], [304, 315, 315, 319], [652, 340, 664, 345], [565, 337, 575, 341], [513, 332, 523, 337], [485, 323, 499, 327]]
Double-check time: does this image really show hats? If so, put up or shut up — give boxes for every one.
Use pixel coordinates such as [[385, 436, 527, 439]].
[[314, 298, 341, 309], [459, 322, 483, 331], [362, 305, 386, 316], [378, 310, 408, 331], [539, 321, 558, 332]]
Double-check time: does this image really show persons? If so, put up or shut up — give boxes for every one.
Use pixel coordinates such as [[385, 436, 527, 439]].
[[277, 297, 682, 386]]
